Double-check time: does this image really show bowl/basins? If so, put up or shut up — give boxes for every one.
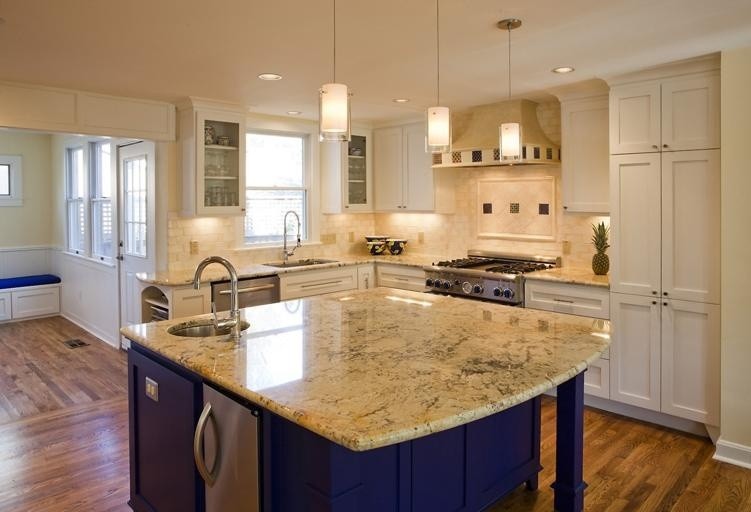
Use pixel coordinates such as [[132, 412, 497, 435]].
[[366, 242, 385, 255], [365, 235, 390, 252], [218, 136, 231, 145], [385, 238, 408, 256]]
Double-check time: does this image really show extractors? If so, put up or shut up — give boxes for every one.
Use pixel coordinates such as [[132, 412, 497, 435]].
[[430, 97, 561, 169]]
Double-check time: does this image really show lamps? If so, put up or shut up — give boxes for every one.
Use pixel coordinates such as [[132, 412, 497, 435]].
[[424, 0, 451, 153], [319, 1, 351, 139], [494, 18, 524, 165]]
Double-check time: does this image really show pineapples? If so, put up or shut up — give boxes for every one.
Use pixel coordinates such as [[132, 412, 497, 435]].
[[588, 219, 610, 275]]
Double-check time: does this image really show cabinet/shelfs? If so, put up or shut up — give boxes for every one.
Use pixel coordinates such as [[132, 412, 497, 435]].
[[136, 271, 212, 323], [376, 123, 457, 214], [559, 97, 609, 213], [264, 258, 358, 300], [524, 265, 608, 411], [0, 275, 61, 324], [608, 153, 720, 304], [377, 244, 462, 292], [605, 69, 721, 150], [608, 291, 721, 443], [320, 128, 375, 213], [181, 108, 249, 217], [121, 338, 200, 512]]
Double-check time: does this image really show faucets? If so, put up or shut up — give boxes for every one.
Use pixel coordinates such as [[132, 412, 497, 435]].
[[282, 210, 302, 264], [194, 255, 240, 340]]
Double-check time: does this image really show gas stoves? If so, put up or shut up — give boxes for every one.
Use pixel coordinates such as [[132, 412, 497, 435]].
[[422, 257, 562, 282]]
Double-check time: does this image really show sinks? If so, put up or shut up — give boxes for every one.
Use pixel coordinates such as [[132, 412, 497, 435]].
[[261, 257, 338, 269], [168, 317, 251, 339]]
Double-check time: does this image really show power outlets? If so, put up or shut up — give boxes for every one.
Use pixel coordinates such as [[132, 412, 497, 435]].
[[562, 241, 570, 254]]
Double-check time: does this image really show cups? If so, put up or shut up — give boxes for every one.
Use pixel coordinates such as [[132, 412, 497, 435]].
[[205, 187, 239, 206]]
